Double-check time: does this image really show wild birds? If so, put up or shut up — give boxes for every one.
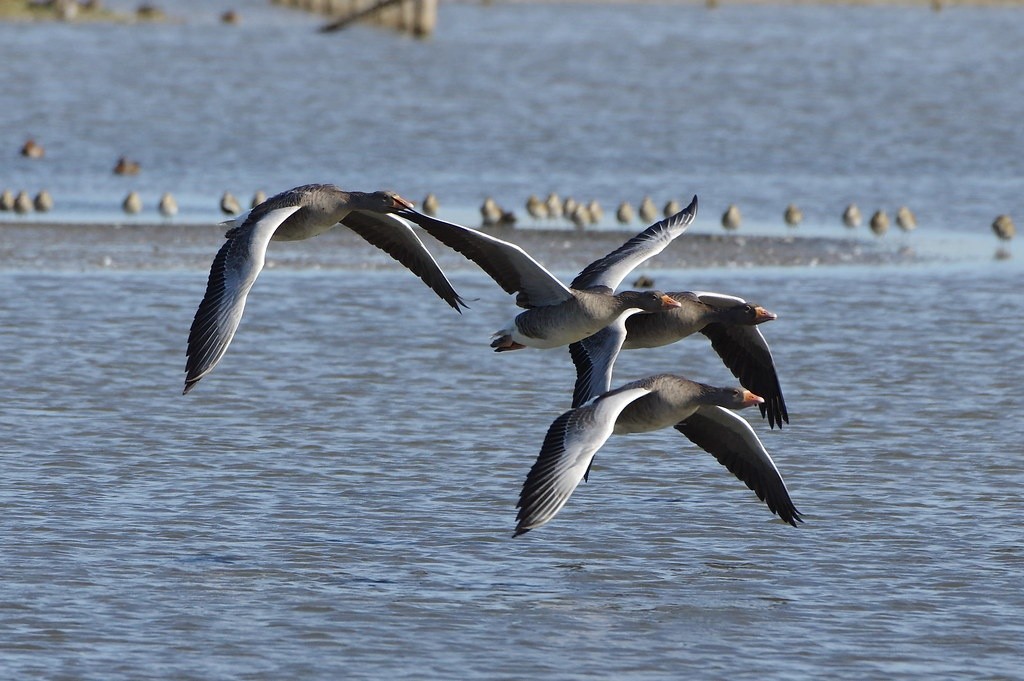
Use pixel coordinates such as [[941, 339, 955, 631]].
[[182, 183, 807, 539]]
